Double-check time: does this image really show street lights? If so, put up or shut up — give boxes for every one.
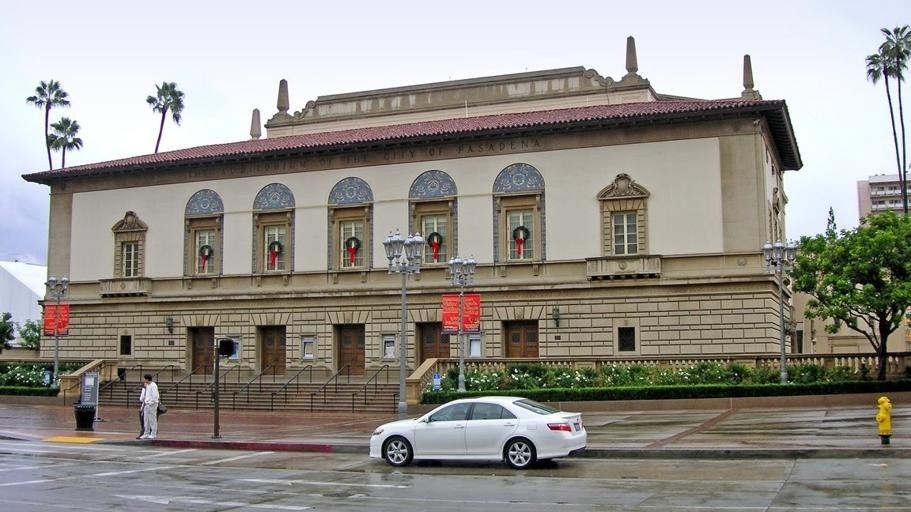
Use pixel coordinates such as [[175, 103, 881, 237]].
[[382, 232, 425, 414], [447, 256, 476, 392], [46, 277, 69, 386], [765, 240, 798, 385]]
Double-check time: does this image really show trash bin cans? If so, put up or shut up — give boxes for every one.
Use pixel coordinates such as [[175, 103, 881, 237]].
[[73, 405, 95, 431]]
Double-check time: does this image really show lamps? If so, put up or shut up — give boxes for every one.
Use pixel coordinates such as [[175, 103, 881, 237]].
[[166, 317, 173, 333], [551, 306, 560, 329]]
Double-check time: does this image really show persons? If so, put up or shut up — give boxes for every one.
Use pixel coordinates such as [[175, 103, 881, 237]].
[[136, 382, 148, 438], [140, 374, 160, 438]]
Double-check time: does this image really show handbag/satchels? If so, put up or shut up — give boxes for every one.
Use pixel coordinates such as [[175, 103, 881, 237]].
[[158, 403, 167, 415]]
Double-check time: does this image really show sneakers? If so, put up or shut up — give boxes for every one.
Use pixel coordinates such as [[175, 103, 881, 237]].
[[136, 434, 156, 439]]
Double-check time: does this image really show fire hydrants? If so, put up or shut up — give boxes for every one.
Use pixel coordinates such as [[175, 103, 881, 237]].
[[876, 396, 892, 444]]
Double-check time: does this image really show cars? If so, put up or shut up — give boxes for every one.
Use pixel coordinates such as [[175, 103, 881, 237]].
[[369, 396, 589, 468]]
